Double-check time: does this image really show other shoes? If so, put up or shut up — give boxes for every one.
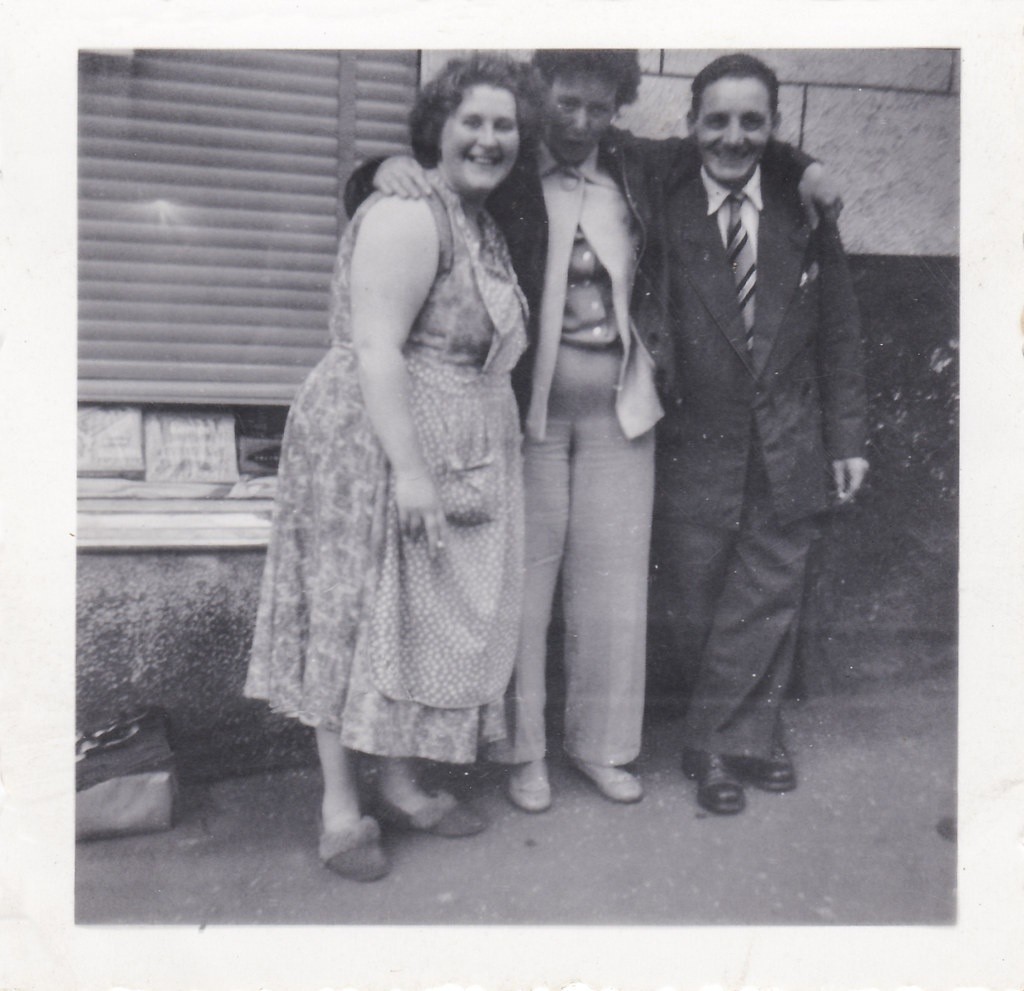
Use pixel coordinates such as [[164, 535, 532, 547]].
[[507, 758, 551, 812], [369, 791, 485, 836], [576, 756, 642, 801], [315, 805, 391, 881]]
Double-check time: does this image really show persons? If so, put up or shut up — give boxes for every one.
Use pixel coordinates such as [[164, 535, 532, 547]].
[[342, 49, 842, 811], [244, 52, 530, 880], [651, 52, 870, 815]]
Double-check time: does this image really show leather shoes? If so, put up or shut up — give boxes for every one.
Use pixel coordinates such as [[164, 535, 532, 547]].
[[745, 757, 795, 791], [682, 745, 744, 813]]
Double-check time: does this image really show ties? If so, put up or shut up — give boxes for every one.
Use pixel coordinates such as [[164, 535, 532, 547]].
[[726, 191, 757, 356]]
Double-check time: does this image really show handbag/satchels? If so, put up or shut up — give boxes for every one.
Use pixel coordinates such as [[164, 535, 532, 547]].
[[76, 705, 178, 841]]
